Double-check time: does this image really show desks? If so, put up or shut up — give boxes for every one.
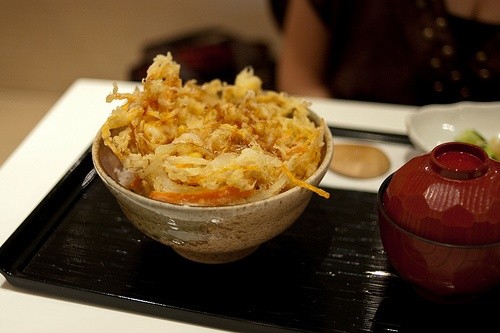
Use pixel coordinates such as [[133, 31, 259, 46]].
[[1, 76, 500, 333]]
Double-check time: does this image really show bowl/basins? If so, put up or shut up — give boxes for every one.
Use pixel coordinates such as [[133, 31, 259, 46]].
[[91, 101, 334, 264], [376, 142, 500, 294], [407, 101, 500, 162]]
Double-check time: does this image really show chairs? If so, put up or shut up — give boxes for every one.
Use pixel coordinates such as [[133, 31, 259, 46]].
[[126, 25, 282, 95]]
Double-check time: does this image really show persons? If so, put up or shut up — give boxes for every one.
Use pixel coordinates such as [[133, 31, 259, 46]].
[[266, 0, 500, 108]]
